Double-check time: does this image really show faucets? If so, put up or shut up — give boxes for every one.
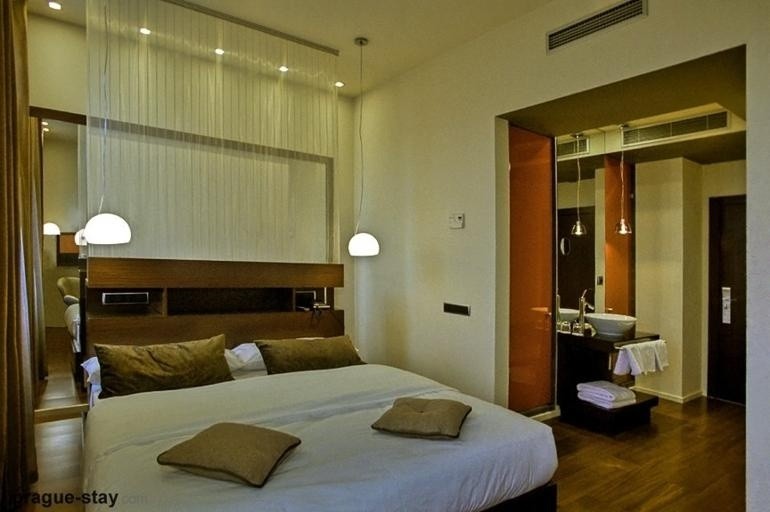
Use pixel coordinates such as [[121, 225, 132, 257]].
[[578, 288, 596, 334], [557, 294, 561, 332]]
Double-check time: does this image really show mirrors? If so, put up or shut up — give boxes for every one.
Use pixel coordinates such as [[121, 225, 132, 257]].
[[28, 105, 333, 424], [556, 129, 606, 333]]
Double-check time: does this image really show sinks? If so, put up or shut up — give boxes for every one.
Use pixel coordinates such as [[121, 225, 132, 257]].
[[587, 312, 638, 337], [559, 305, 579, 324]]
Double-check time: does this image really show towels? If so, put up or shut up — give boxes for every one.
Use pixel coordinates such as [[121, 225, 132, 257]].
[[634, 342, 656, 376], [647, 339, 670, 371], [613, 344, 645, 376], [576, 380, 636, 409]]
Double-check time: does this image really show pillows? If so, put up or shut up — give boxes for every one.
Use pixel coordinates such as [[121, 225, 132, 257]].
[[93, 334, 236, 399], [371, 397, 472, 440], [253, 334, 368, 375], [230, 336, 359, 372], [80, 349, 246, 385], [157, 423, 301, 489]]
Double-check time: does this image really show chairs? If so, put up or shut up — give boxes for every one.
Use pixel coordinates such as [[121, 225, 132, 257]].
[[57, 276, 80, 304]]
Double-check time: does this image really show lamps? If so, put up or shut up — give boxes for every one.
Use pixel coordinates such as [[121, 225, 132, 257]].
[[614, 122, 632, 235], [43, 222, 61, 236], [570, 131, 587, 236], [348, 36, 379, 257], [82, 0, 131, 246]]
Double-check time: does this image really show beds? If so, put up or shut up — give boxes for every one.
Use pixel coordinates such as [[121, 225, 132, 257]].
[[55, 232, 87, 404], [82, 257, 559, 512]]
